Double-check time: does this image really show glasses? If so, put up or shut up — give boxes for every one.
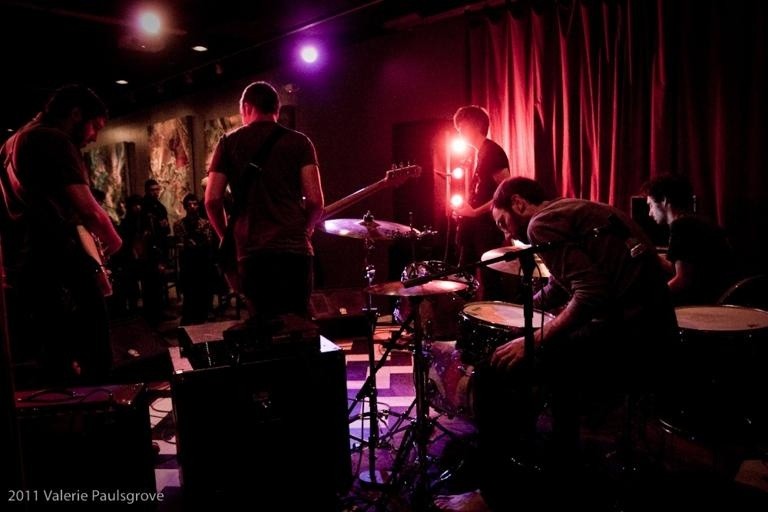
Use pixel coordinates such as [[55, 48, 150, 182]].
[[498, 222, 507, 233]]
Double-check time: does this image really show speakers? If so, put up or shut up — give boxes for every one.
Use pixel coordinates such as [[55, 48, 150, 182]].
[[165, 331, 354, 512]]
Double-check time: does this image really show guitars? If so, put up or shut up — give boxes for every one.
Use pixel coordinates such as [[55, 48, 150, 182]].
[[212, 162, 424, 301], [61, 213, 114, 301]]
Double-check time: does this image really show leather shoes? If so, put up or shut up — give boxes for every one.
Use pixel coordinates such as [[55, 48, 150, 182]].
[[147, 316, 161, 328], [161, 312, 176, 320]]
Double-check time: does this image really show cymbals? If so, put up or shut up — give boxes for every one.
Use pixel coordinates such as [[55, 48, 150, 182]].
[[362, 276, 474, 299], [312, 210, 428, 244], [480, 242, 557, 279]]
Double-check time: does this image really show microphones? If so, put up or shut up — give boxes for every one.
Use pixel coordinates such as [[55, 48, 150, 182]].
[[609, 213, 638, 250]]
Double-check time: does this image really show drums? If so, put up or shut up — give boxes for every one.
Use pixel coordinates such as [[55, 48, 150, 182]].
[[408, 341, 485, 421], [671, 302, 767, 346], [396, 270, 480, 342], [455, 298, 560, 374], [646, 408, 756, 510]]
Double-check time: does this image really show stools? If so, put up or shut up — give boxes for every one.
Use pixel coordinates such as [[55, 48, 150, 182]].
[[589, 388, 660, 512]]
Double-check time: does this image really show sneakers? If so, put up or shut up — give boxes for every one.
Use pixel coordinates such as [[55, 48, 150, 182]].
[[219, 295, 230, 307], [239, 292, 246, 308]]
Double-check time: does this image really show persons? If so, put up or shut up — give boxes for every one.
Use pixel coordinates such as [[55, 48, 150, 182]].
[[172, 194, 214, 325], [646, 177, 741, 308], [1, 86, 121, 389], [138, 179, 170, 239], [204, 80, 323, 328], [451, 106, 510, 269], [492, 176, 679, 368]]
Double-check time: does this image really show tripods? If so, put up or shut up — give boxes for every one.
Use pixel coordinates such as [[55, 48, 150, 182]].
[[470, 214, 616, 511], [348, 300, 482, 512]]
